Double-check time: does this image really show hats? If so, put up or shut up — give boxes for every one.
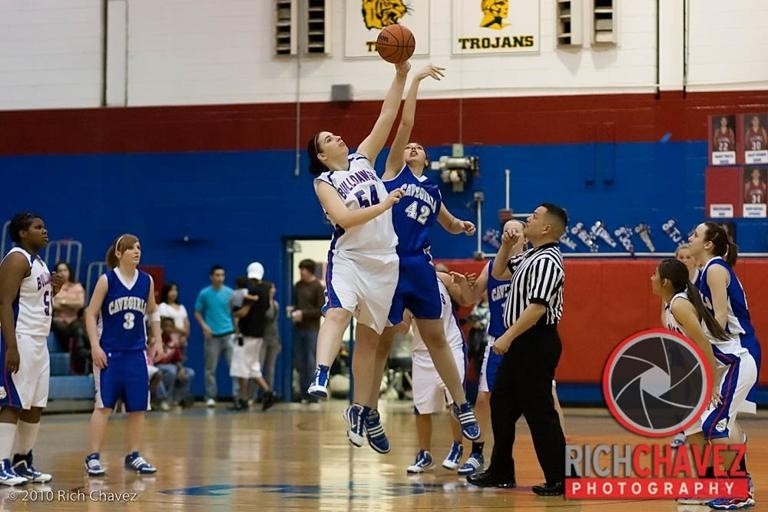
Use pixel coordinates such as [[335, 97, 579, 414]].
[[245, 260, 266, 282]]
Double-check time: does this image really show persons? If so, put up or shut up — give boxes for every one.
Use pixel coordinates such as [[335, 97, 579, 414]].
[[744, 116, 768, 151], [713, 117, 735, 151], [0, 61, 581, 496], [743, 167, 766, 204], [650, 220, 761, 510]]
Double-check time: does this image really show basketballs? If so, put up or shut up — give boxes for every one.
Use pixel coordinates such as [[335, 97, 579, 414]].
[[376, 25, 416, 65]]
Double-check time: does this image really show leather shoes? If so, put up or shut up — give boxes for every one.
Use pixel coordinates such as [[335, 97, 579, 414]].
[[529, 478, 568, 498], [464, 471, 518, 489]]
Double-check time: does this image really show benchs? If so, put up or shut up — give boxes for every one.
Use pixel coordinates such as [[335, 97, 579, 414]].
[[41, 325, 98, 411]]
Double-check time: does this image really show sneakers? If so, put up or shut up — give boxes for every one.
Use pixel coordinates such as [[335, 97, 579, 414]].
[[83, 452, 105, 477], [124, 451, 158, 474], [455, 451, 485, 475], [450, 401, 482, 441], [0, 458, 29, 488], [362, 409, 392, 454], [148, 389, 323, 411], [10, 450, 53, 483], [305, 363, 331, 400], [405, 449, 438, 474], [341, 401, 373, 448], [674, 472, 757, 510], [442, 439, 464, 471], [669, 432, 688, 449]]
